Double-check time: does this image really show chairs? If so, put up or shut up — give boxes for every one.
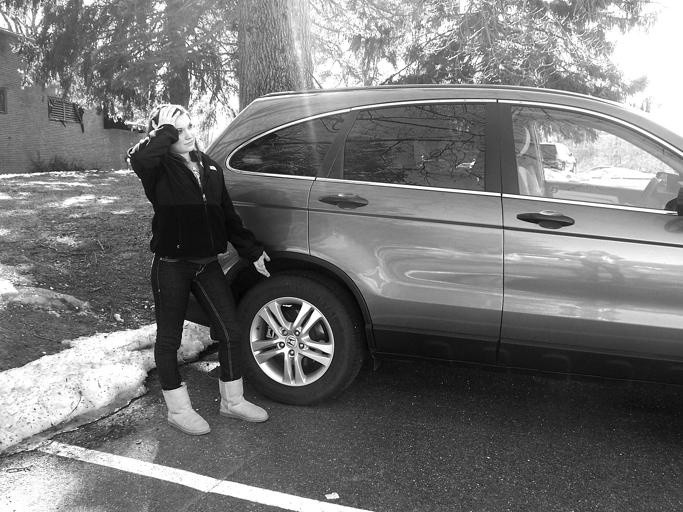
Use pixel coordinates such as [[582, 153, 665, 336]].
[[513, 124, 543, 197]]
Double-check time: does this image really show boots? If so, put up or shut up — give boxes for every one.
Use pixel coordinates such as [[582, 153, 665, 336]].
[[219, 375, 270, 424], [162, 381, 210, 437]]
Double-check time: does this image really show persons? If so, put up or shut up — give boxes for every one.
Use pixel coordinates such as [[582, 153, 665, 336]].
[[127, 103, 272, 436]]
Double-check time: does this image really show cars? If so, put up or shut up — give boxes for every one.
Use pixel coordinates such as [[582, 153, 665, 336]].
[[184, 84, 683, 406]]
